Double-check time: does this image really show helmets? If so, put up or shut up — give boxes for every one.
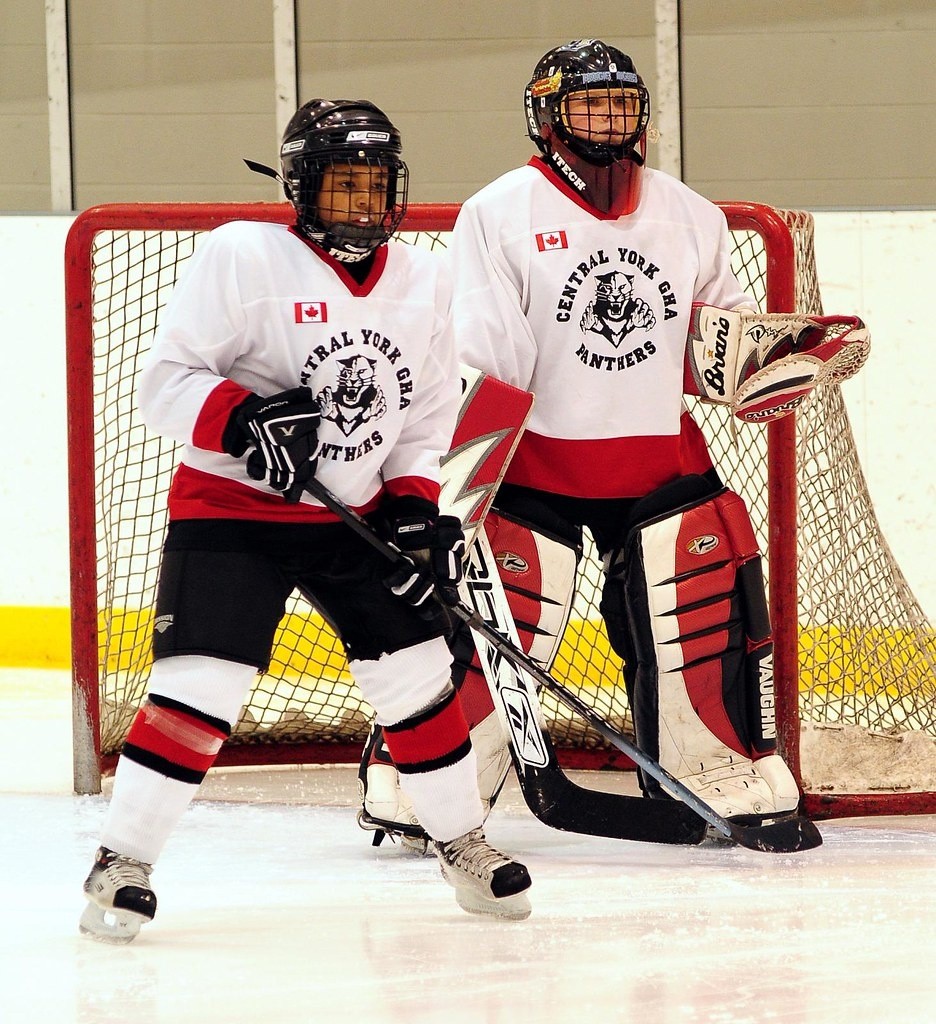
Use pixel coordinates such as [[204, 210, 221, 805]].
[[280, 98, 402, 211], [524, 38, 650, 143]]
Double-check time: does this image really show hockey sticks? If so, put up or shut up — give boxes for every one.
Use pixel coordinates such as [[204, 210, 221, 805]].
[[453, 528, 711, 848], [302, 475, 822, 854]]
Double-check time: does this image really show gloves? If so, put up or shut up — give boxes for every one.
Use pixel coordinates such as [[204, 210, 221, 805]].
[[383, 495, 465, 620], [222, 387, 321, 504]]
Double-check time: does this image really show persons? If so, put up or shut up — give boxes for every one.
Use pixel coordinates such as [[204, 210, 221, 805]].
[[77, 95, 536, 920], [355, 37, 823, 831]]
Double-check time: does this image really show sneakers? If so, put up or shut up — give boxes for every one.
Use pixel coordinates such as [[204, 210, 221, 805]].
[[435, 825, 532, 920], [79, 846, 156, 945]]
